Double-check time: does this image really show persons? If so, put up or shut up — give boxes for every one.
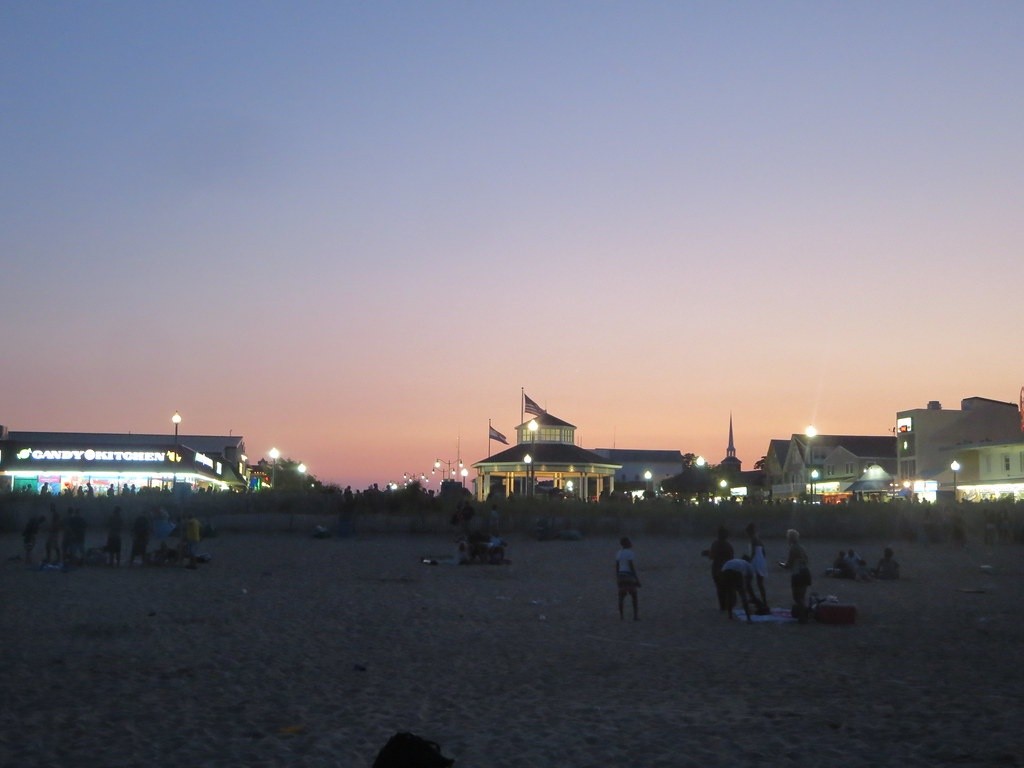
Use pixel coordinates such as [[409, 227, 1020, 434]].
[[834, 549, 900, 581], [23, 502, 200, 571], [615, 537, 641, 621], [709, 523, 767, 624], [778, 529, 811, 624], [457, 500, 510, 565]]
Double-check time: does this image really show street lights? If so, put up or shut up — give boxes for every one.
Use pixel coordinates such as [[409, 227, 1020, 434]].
[[811, 470, 819, 503], [805, 426, 818, 503], [404, 472, 425, 481], [527, 420, 538, 494], [950, 460, 960, 500], [435, 458, 463, 481], [644, 470, 653, 490], [171, 414, 182, 489], [460, 469, 468, 489], [523, 453, 532, 495], [269, 449, 280, 489], [432, 467, 456, 480]]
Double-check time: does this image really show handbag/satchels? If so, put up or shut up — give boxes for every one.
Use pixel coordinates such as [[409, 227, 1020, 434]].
[[791, 605, 807, 618]]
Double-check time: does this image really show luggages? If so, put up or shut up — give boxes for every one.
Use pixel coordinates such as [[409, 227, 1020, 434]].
[[816, 601, 856, 625]]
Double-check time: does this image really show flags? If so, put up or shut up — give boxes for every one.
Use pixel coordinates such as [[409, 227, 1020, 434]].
[[490, 427, 509, 445], [525, 394, 547, 421]]
